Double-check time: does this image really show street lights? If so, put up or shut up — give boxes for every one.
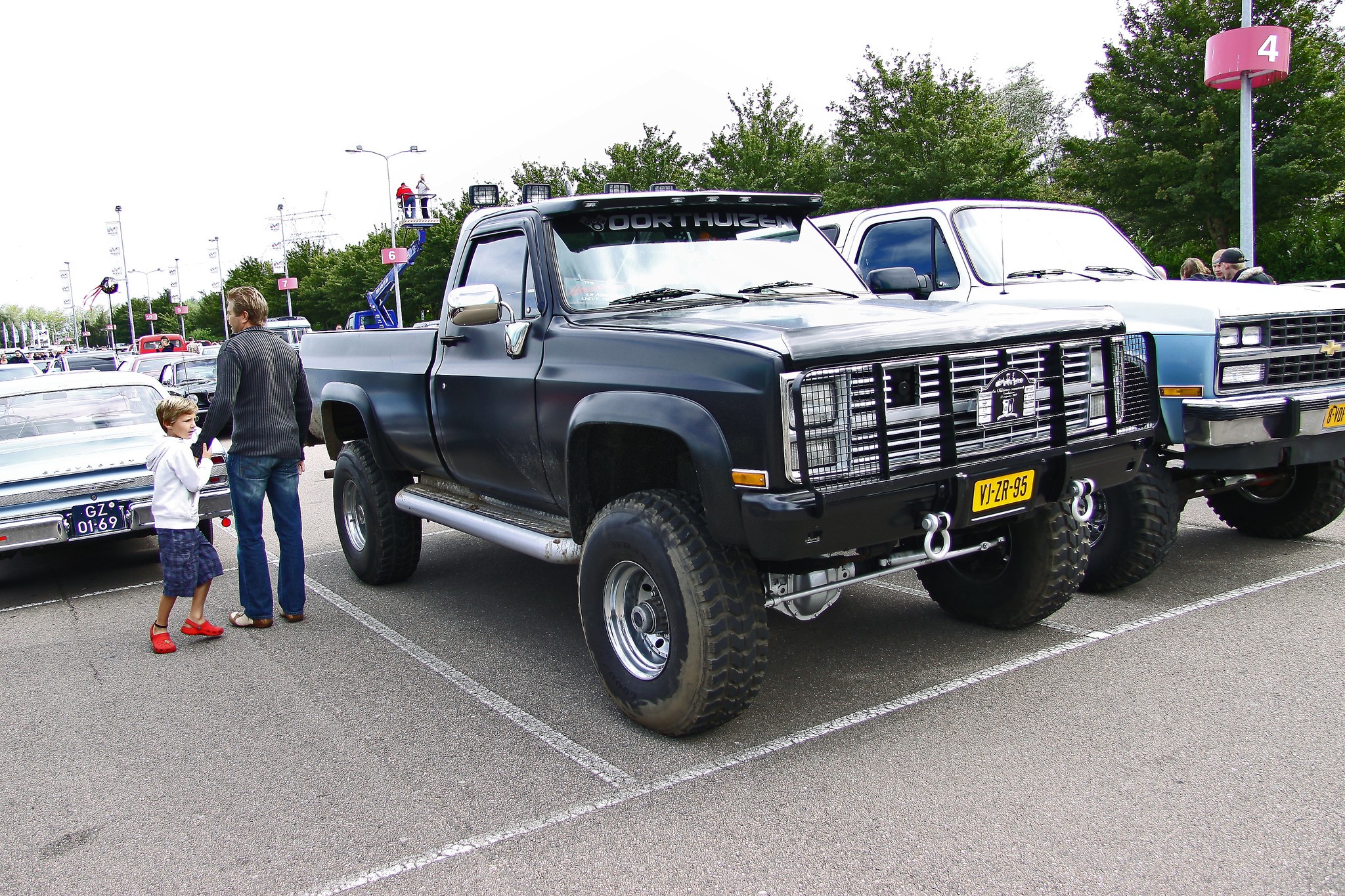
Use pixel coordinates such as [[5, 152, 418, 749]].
[[46, 321, 64, 346], [208, 237, 230, 341], [55, 316, 70, 344], [277, 205, 292, 317], [114, 206, 137, 353], [64, 262, 80, 353], [346, 145, 427, 328], [100, 329, 110, 348], [128, 269, 164, 344], [74, 306, 94, 347], [175, 259, 186, 343]]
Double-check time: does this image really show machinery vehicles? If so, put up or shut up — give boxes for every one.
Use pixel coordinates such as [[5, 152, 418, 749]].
[[345, 193, 441, 331]]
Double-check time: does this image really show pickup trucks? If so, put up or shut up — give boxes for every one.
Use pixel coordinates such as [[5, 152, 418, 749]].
[[304, 183, 1345, 740]]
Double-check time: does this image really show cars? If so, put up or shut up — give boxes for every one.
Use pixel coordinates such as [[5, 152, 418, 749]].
[[0, 318, 312, 553]]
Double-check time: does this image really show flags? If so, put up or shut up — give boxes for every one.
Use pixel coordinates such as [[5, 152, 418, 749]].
[[23, 322, 27, 341], [33, 321, 38, 340], [14, 325, 19, 343], [5, 324, 9, 342]]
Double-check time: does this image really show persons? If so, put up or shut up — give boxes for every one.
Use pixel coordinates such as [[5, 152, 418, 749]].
[[1179, 258, 1221, 281], [416, 174, 430, 219], [186, 337, 200, 354], [146, 396, 224, 653], [214, 340, 225, 345], [1212, 249, 1231, 281], [1213, 248, 1270, 284], [10, 350, 29, 364], [396, 183, 416, 219], [28, 345, 74, 360], [0, 353, 10, 364], [155, 336, 175, 352], [189, 287, 312, 628]]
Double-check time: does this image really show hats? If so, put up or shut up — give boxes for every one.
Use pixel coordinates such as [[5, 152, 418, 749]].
[[1213, 247, 1250, 265]]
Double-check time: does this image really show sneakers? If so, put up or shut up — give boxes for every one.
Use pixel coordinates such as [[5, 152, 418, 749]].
[[229, 610, 274, 628], [280, 607, 303, 622]]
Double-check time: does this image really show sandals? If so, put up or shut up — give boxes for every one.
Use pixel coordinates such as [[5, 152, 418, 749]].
[[150, 623, 176, 653], [181, 618, 224, 636]]
